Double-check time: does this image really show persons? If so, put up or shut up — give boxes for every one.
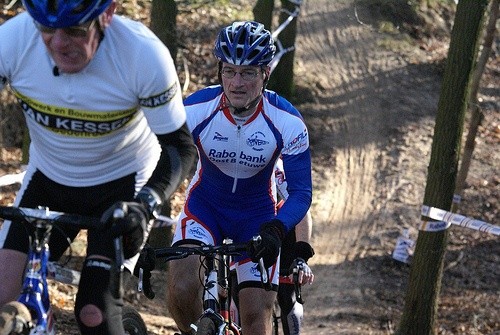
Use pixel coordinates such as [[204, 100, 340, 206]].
[[166, 22, 314, 335], [0, 0, 194, 335]]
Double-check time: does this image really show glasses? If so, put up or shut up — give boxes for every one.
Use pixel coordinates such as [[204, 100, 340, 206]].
[[32, 20, 96, 37], [218, 68, 262, 80]]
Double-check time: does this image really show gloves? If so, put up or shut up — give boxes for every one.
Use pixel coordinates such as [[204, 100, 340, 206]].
[[100, 201, 150, 259], [251, 219, 282, 270]]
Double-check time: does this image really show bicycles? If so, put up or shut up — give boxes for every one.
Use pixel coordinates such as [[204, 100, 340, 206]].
[[0, 204, 148, 335], [272, 266, 306, 335], [137, 235, 271, 335]]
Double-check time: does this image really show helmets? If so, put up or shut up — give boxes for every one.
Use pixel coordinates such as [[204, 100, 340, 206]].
[[212, 21, 276, 66], [22, 0, 113, 27]]
